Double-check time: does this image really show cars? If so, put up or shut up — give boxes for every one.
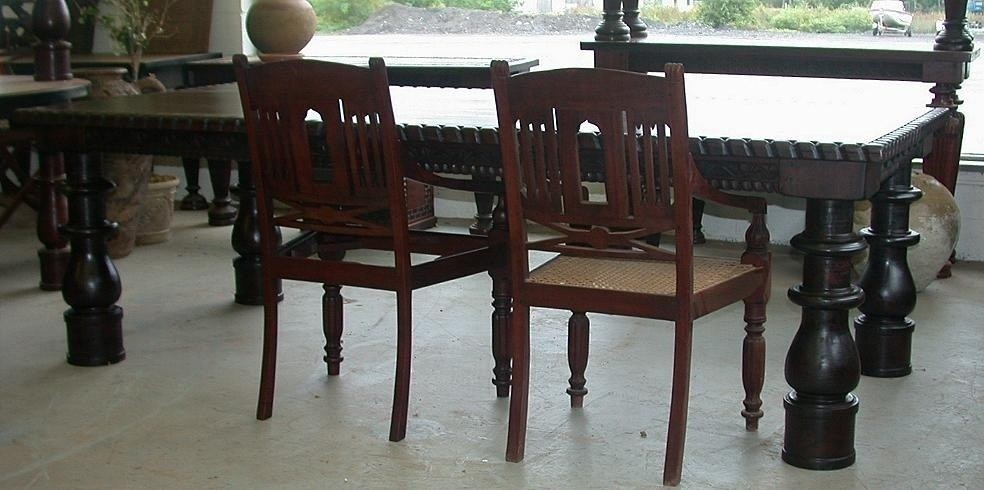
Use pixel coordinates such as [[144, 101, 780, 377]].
[[966, 0, 984, 17]]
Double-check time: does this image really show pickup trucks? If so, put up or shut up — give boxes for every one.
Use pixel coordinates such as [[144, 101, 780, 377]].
[[869, 0, 904, 20]]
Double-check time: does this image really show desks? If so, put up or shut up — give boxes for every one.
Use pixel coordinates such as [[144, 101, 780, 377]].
[[574, 33, 983, 278]]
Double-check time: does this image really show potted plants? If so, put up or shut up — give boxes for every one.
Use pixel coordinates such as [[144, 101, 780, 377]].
[[73, 0, 182, 246]]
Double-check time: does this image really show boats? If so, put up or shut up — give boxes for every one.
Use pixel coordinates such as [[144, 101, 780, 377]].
[[868, 8, 913, 37]]
